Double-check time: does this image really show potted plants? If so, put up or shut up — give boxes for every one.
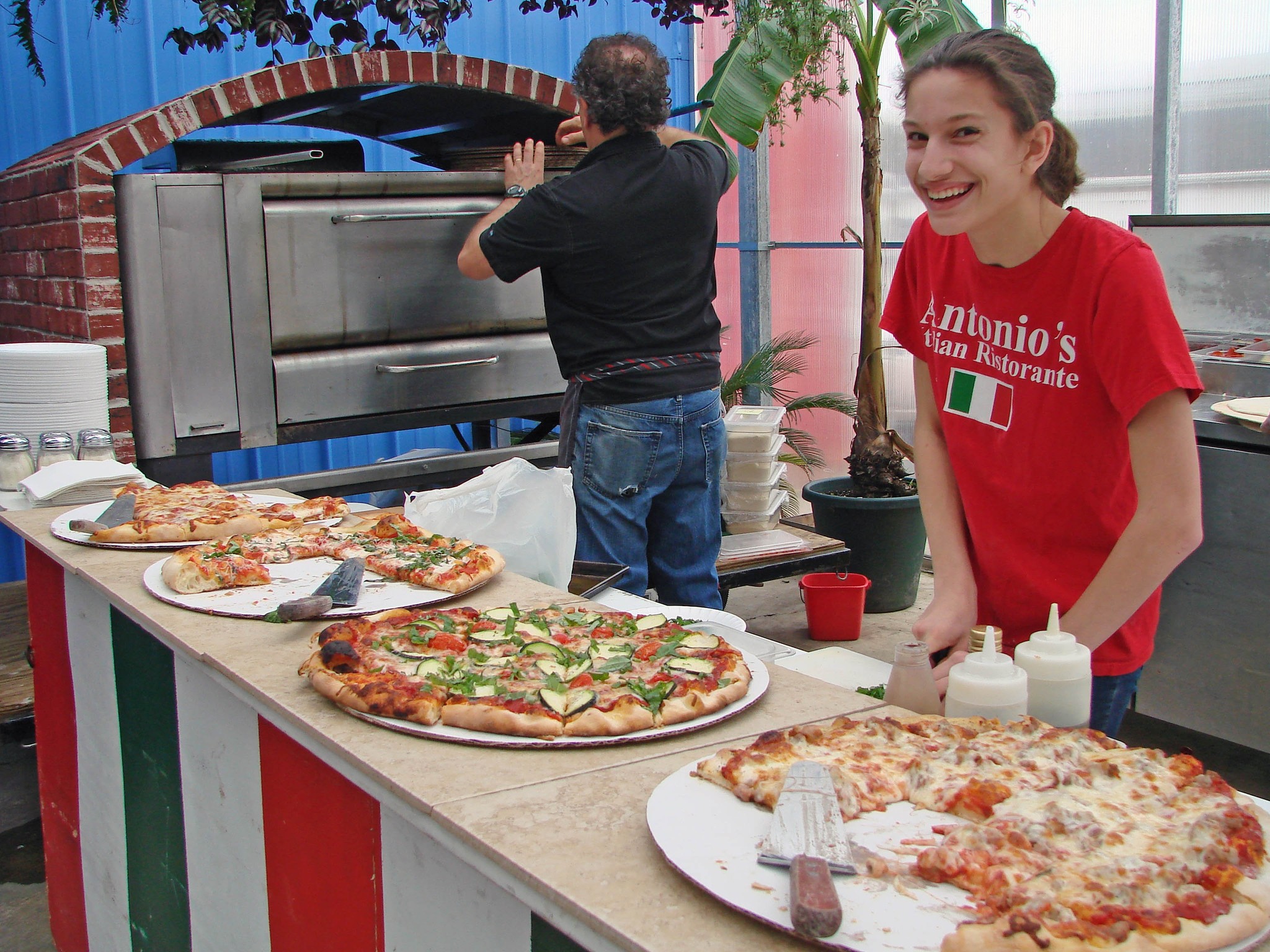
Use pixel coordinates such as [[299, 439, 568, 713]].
[[694, 0, 985, 614]]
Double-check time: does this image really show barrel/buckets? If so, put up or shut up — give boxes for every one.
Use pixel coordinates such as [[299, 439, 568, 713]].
[[798, 573, 872, 641]]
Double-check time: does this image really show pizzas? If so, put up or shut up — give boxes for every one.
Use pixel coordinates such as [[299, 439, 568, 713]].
[[159, 513, 507, 594], [692, 712, 1270, 952], [299, 601, 752, 735], [88, 481, 353, 543]]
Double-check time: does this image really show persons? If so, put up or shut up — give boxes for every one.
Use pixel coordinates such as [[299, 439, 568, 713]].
[[457, 31, 727, 611], [878, 27, 1203, 739]]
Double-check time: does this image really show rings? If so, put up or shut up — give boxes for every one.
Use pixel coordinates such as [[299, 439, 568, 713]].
[[513, 158, 522, 161]]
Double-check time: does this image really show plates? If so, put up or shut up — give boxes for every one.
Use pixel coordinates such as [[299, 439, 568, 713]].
[[0, 342, 110, 470]]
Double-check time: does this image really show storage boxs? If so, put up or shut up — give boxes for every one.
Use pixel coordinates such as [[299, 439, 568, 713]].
[[721, 404, 791, 535]]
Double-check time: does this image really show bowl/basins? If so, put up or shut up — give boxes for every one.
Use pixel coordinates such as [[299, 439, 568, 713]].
[[1234, 340, 1270, 364], [719, 405, 788, 535]]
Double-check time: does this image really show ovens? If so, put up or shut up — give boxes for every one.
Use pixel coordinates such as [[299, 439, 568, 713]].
[[113, 171, 575, 462]]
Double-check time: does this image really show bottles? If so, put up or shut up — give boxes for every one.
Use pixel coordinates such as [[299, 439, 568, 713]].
[[967, 626, 1003, 653], [883, 641, 943, 717], [1013, 602, 1092, 728], [945, 627, 1028, 724], [0, 428, 117, 493]]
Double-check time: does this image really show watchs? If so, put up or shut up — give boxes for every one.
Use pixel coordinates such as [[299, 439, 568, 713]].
[[503, 185, 528, 199]]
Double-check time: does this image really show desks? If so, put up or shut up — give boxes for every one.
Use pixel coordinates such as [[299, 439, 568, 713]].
[[714, 518, 853, 608], [0, 485, 1270, 952]]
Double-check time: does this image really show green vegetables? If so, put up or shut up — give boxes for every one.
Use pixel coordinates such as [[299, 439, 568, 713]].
[[667, 616, 705, 625], [856, 683, 888, 700]]
